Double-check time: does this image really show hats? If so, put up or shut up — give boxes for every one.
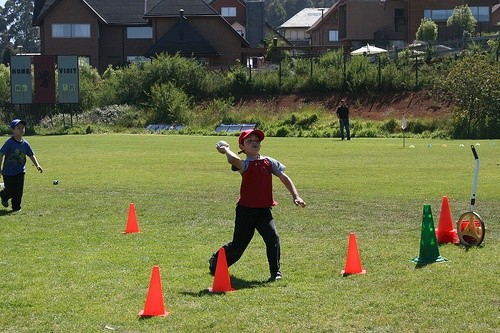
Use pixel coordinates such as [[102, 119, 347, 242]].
[[10, 119, 27, 128], [238, 129, 265, 144]]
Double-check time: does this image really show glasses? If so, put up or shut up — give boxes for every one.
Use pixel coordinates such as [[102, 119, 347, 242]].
[[244, 138, 260, 144]]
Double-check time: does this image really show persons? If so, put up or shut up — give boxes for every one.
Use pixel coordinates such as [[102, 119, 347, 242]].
[[336, 100, 351, 141], [0, 119, 43, 210], [209, 130, 306, 279]]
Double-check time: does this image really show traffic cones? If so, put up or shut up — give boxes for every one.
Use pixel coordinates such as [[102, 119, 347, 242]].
[[341, 233, 366, 275], [208, 247, 235, 293], [121, 203, 140, 233], [137, 265, 170, 316], [409, 203, 452, 263], [435, 196, 460, 243]]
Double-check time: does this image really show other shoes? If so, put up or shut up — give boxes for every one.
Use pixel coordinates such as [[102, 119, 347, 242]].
[[0, 191, 8, 207], [209, 252, 215, 276], [13, 206, 21, 212], [271, 270, 282, 280]]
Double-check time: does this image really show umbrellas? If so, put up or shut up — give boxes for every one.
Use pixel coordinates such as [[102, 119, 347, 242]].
[[350, 45, 387, 56]]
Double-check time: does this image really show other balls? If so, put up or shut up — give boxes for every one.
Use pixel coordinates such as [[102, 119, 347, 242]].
[[53, 180, 59, 185], [216, 141, 229, 154]]
